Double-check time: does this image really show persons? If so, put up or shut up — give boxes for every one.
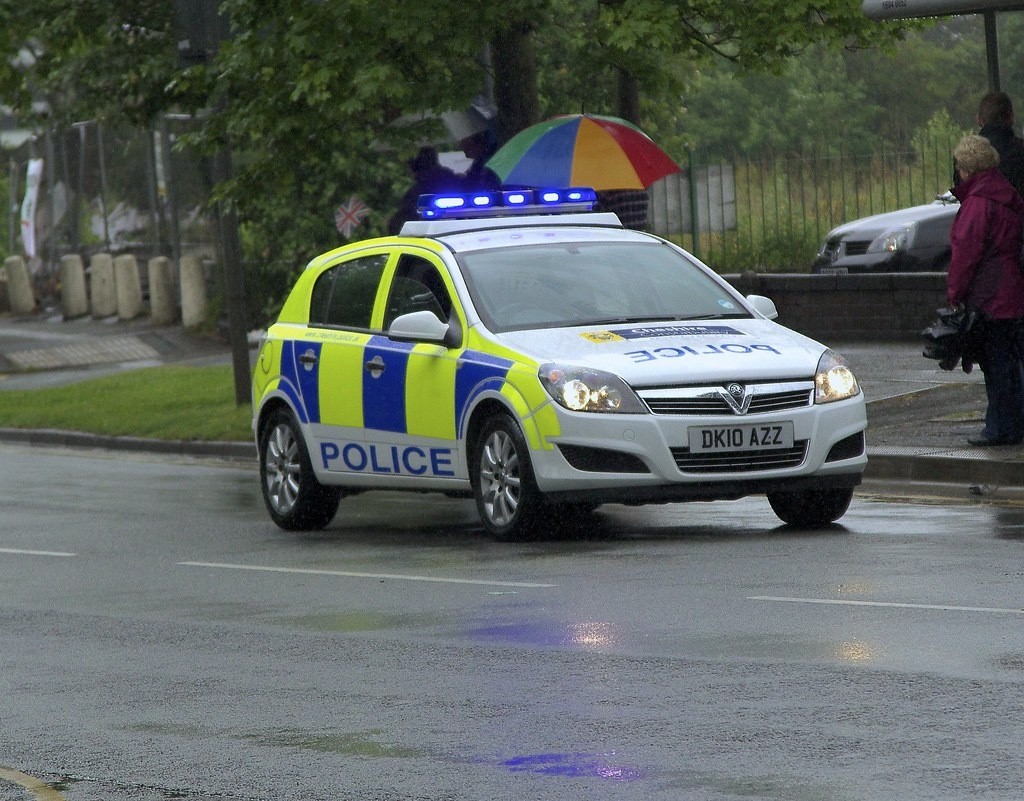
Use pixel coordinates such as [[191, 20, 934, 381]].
[[387, 132, 504, 235], [947, 135, 1024, 446], [596, 186, 649, 231], [951, 92, 1024, 192]]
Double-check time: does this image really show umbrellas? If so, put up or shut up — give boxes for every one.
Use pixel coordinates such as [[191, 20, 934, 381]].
[[368, 102, 499, 150], [485, 111, 682, 191], [923, 303, 984, 373]]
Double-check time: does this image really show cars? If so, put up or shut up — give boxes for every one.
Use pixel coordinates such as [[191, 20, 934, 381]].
[[251, 185, 869, 543], [807, 190, 962, 275]]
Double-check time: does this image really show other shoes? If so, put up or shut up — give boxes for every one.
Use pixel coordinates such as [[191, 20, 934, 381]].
[[967, 435, 996, 447]]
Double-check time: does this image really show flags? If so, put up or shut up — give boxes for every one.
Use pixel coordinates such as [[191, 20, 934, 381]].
[[333, 197, 371, 240]]
